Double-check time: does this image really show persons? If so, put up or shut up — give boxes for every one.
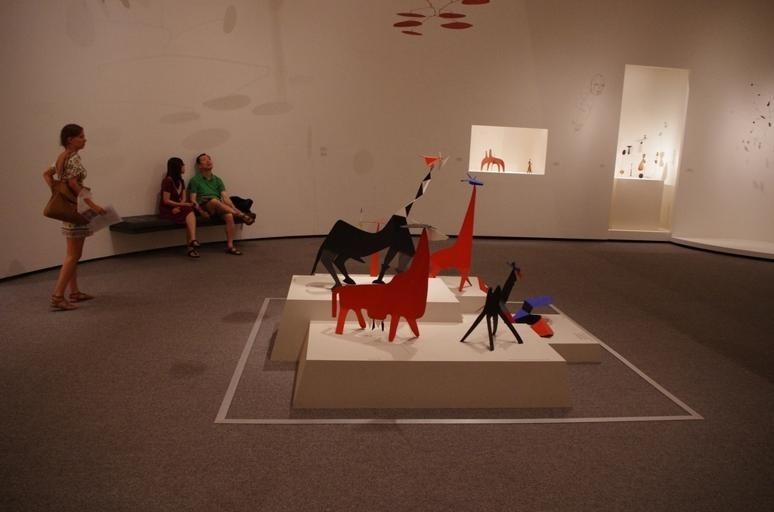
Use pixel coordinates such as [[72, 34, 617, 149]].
[[159, 158, 201, 258], [187, 153, 254, 255], [43, 123, 106, 309]]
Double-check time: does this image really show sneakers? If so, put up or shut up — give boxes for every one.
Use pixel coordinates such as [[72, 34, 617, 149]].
[[227, 247, 242, 255], [238, 212, 254, 225]]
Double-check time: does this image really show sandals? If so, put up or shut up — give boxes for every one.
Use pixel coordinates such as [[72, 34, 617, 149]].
[[49, 294, 79, 309], [69, 292, 94, 303]]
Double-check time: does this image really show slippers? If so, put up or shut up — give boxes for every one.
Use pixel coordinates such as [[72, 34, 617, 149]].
[[188, 249, 200, 257], [187, 240, 201, 247]]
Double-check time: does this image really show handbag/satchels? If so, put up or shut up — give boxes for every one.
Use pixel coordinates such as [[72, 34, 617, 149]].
[[230, 196, 253, 213], [43, 180, 91, 226]]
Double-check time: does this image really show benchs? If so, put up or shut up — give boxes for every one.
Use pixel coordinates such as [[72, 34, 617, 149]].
[[109, 213, 243, 235]]
